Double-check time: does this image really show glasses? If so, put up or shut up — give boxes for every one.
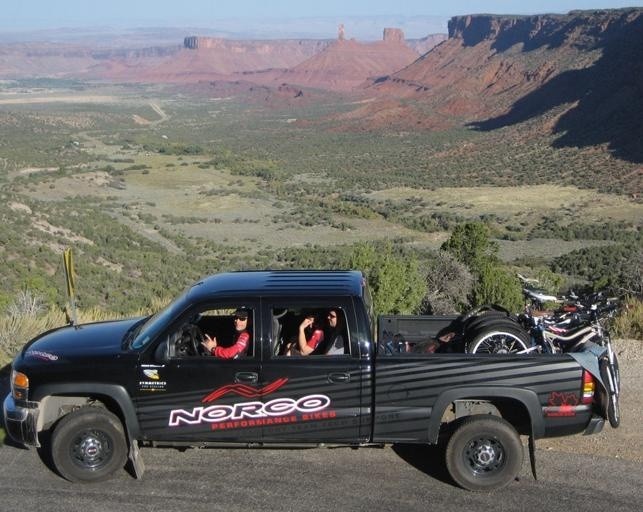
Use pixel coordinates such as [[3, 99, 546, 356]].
[[234, 317, 246, 321], [329, 313, 335, 318]]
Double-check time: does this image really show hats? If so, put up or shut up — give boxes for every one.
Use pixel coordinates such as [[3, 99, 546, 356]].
[[231, 308, 252, 317]]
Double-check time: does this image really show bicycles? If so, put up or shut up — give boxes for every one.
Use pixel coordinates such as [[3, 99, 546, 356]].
[[456, 270, 623, 430]]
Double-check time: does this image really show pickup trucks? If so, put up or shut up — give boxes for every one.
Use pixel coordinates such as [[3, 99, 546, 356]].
[[0, 265, 624, 495]]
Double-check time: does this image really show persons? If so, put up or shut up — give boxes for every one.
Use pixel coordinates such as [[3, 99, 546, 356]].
[[284, 310, 345, 357], [200, 308, 250, 359]]
